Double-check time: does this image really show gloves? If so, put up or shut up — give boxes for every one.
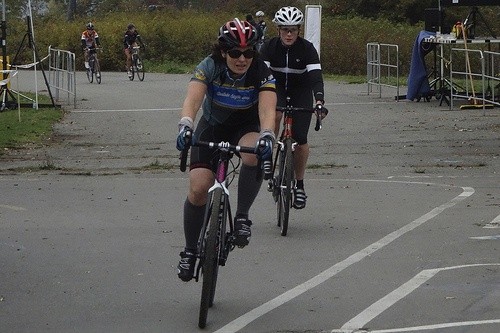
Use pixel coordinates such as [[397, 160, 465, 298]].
[[176, 125, 194, 151], [256, 135, 275, 160]]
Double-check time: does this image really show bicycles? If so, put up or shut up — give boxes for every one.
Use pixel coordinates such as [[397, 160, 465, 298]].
[[127, 46, 144, 81], [264, 102, 323, 236], [86, 47, 101, 84], [178, 131, 274, 329]]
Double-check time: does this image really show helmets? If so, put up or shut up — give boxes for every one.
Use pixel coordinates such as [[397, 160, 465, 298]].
[[217, 18, 258, 49], [256, 11, 264, 17], [126, 24, 136, 30], [272, 7, 304, 26], [87, 23, 95, 29]]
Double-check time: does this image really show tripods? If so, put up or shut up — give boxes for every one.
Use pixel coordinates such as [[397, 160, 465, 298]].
[[460, 7, 496, 39], [427, 42, 467, 93]]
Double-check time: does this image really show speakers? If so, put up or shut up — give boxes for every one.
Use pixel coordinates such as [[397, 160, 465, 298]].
[[450, 0, 500, 6]]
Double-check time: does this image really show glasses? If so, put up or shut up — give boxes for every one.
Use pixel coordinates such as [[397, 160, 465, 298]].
[[226, 49, 254, 59], [281, 27, 298, 32]]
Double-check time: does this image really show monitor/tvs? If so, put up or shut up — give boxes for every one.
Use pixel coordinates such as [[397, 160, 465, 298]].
[[424, 8, 447, 33]]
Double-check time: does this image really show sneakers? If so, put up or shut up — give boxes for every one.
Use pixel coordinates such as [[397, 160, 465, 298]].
[[264, 160, 272, 179], [177, 248, 197, 282], [293, 188, 306, 209], [234, 213, 252, 246]]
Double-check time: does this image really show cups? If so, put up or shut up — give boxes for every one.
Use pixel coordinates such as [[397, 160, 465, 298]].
[[436, 32, 456, 38]]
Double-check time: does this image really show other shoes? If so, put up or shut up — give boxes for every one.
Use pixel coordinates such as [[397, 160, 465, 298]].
[[85, 62, 90, 68], [97, 74, 100, 77], [128, 71, 132, 77]]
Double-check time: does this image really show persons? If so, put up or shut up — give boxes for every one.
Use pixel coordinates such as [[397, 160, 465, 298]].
[[246, 13, 263, 50], [80, 22, 102, 69], [256, 8, 329, 208], [123, 24, 144, 77], [255, 11, 266, 52], [174, 19, 278, 281]]
